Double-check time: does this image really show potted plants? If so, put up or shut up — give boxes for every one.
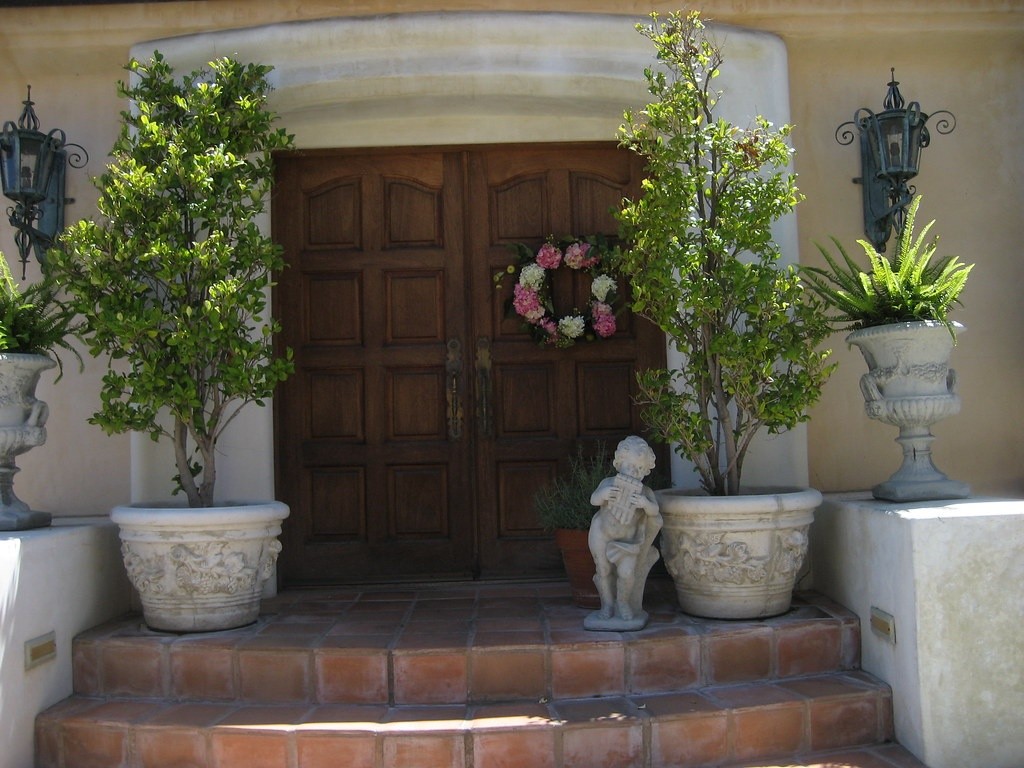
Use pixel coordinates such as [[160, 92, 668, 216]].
[[529, 439, 617, 608], [48, 52, 302, 632], [0, 256, 98, 532], [618, 10, 838, 619], [801, 193, 975, 500]]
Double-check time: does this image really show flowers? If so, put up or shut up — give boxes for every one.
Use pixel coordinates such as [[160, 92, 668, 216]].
[[512, 232, 626, 343]]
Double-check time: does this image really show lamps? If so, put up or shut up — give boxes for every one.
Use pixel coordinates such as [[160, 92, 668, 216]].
[[1, 85, 90, 280], [843, 64, 949, 251]]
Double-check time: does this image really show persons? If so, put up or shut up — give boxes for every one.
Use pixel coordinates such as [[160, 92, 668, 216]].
[[588, 436, 659, 620]]
